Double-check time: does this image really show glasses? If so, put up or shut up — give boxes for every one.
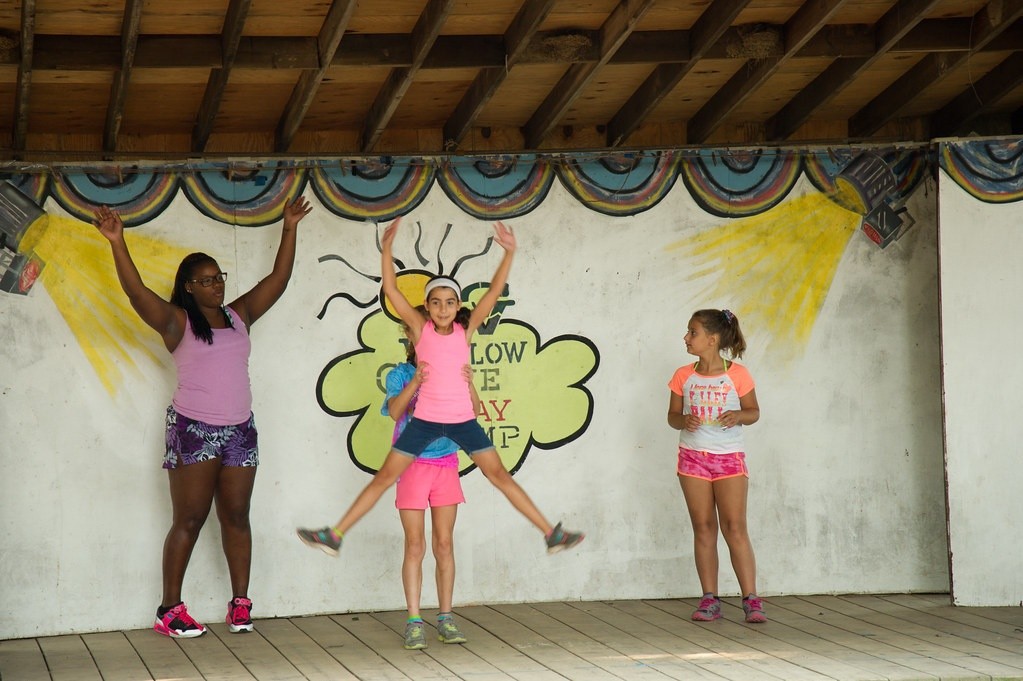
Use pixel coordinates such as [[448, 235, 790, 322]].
[[188, 273, 227, 288]]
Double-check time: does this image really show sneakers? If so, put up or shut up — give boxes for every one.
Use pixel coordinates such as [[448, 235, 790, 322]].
[[742, 593, 767, 622], [153, 601, 207, 638], [437, 619, 467, 643], [544, 522, 585, 555], [404, 622, 428, 650], [225, 597, 254, 633], [296, 526, 343, 556], [691, 592, 721, 621]]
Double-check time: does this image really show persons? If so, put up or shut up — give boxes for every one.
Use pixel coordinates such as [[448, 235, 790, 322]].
[[668, 310, 768, 622], [91, 193, 314, 639], [298, 215, 586, 554], [380, 305, 468, 649]]
